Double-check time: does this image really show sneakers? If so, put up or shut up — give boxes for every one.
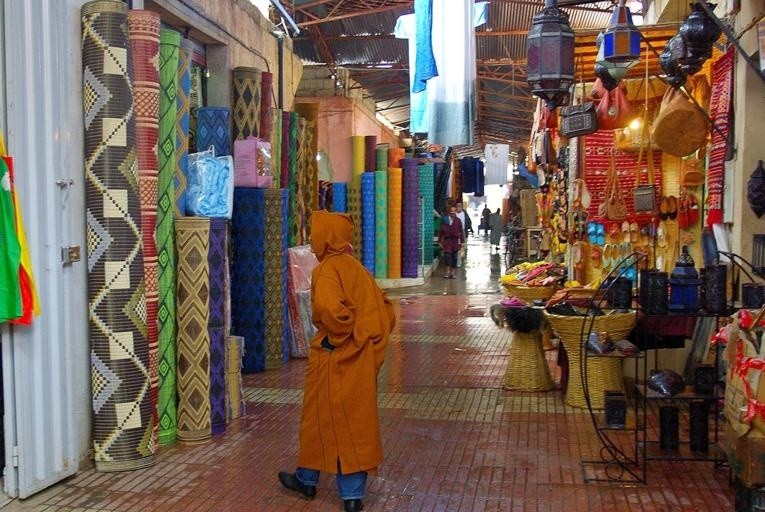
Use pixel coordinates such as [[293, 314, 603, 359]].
[[588, 222, 666, 269], [659, 194, 699, 230]]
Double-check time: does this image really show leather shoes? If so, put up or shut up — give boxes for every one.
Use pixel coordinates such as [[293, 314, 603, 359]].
[[279, 472, 317, 499], [345, 498, 363, 511]]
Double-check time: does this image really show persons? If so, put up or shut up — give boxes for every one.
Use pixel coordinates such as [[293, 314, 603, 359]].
[[437, 206, 466, 279], [489, 208, 504, 250], [482, 204, 491, 235], [456, 203, 474, 267], [276, 208, 395, 512]]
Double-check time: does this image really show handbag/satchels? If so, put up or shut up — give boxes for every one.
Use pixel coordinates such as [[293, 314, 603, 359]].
[[599, 201, 626, 222], [589, 76, 632, 129], [632, 187, 657, 211], [559, 101, 596, 138], [681, 158, 707, 188]]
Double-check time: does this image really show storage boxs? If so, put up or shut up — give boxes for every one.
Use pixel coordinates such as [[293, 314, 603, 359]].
[[717, 306, 764, 489]]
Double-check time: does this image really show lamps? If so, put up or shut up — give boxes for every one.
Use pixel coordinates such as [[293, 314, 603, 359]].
[[658, 2, 723, 89], [524, 1, 578, 112], [594, 0, 643, 95]]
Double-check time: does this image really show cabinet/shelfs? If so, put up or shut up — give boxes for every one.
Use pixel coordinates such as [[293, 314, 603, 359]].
[[573, 249, 764, 486]]
